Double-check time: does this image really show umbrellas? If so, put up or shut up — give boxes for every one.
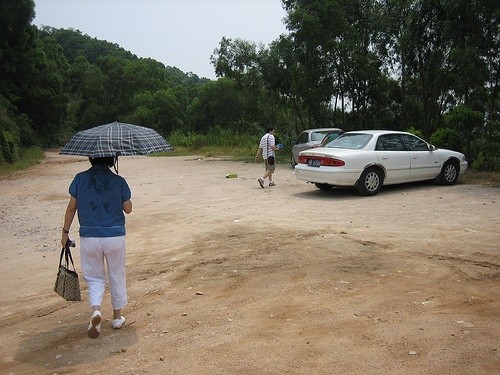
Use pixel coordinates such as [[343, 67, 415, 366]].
[[59, 121, 174, 176]]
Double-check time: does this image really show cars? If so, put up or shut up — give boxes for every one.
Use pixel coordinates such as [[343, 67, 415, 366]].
[[290, 127, 347, 169], [292, 130, 469, 196]]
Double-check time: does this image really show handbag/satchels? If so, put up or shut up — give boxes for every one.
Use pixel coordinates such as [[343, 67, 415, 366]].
[[268, 155, 274, 164], [54, 238, 81, 301]]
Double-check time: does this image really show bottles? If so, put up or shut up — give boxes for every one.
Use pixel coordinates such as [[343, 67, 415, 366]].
[[278, 145, 281, 150], [226, 174, 238, 178]]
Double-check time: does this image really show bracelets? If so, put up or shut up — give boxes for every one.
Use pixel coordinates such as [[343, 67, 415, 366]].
[[63, 228, 69, 233]]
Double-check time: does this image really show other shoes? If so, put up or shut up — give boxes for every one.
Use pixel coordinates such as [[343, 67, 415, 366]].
[[269, 181, 276, 185], [113, 315, 126, 328], [88, 310, 102, 338], [258, 177, 264, 187]]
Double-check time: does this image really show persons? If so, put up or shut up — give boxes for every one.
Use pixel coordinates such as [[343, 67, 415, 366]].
[[61, 156, 133, 339], [255, 127, 281, 188]]
